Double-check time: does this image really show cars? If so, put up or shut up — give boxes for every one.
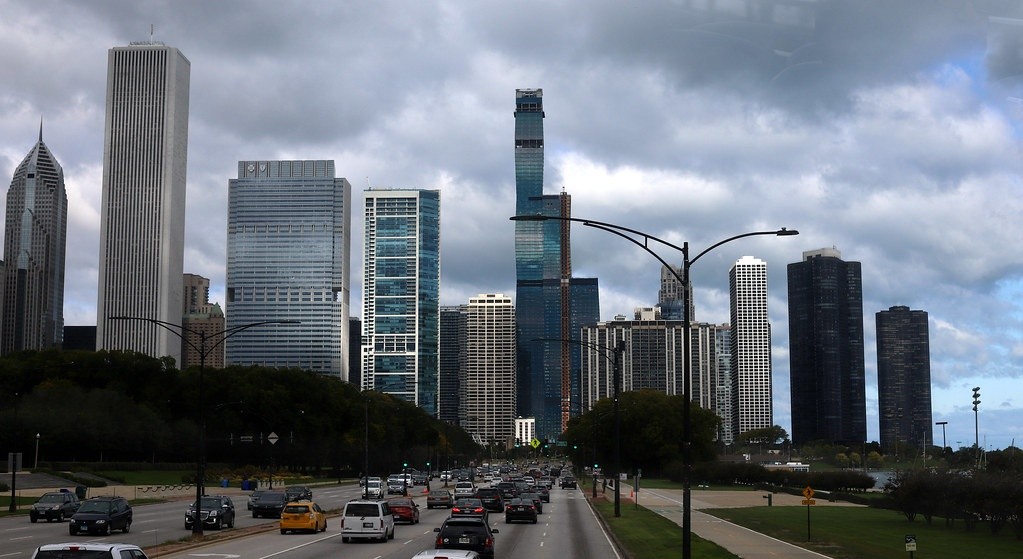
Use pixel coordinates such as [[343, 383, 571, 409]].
[[248, 486, 313, 510], [279, 502, 327, 534], [359, 463, 577, 524], [253, 492, 287, 517]]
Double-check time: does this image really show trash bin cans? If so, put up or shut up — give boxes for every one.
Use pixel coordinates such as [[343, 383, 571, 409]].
[[221, 479, 229, 487], [248, 481, 257, 490], [75, 485, 87, 499], [242, 480, 249, 490]]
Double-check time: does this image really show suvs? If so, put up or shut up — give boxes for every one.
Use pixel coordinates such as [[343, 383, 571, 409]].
[[32, 542, 149, 559], [30, 491, 80, 522], [411, 548, 481, 559], [340, 498, 395, 543], [184, 493, 235, 531], [69, 495, 133, 537], [433, 516, 500, 559]]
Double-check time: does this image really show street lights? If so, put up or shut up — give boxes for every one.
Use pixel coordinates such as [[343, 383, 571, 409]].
[[936, 421, 947, 448], [528, 336, 625, 518], [558, 399, 598, 498], [509, 210, 799, 559], [107, 314, 301, 536], [336, 378, 396, 499]]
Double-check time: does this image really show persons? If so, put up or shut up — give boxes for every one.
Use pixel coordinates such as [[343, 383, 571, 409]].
[[609, 479, 613, 487], [601, 479, 607, 493]]
